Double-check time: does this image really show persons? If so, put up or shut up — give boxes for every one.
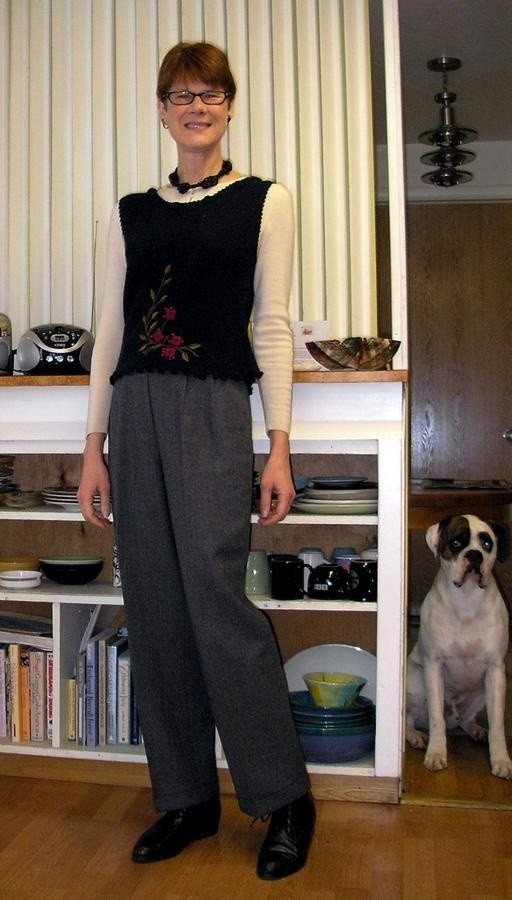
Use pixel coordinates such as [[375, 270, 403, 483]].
[[76, 42, 313, 881]]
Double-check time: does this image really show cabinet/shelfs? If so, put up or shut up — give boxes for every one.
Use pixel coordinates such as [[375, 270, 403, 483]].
[[1, 369, 410, 806]]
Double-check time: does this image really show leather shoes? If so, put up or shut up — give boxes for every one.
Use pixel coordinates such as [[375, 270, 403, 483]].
[[132, 802, 219, 864], [255, 798, 316, 881]]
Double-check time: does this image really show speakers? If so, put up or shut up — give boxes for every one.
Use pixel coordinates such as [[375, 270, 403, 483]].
[[0, 312, 13, 375]]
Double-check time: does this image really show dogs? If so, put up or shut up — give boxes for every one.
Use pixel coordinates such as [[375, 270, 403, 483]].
[[406, 511, 512, 780]]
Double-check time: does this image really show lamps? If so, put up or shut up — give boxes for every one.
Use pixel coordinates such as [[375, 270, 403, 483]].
[[418, 57, 479, 187]]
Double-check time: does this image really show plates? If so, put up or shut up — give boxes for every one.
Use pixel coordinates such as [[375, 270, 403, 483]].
[[284, 644, 376, 703], [252, 471, 377, 513], [42, 487, 113, 513]]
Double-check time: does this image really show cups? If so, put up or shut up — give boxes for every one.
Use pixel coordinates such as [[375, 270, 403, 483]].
[[245, 547, 378, 602]]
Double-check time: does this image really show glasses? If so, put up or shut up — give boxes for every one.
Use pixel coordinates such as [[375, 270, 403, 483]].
[[159, 91, 231, 105]]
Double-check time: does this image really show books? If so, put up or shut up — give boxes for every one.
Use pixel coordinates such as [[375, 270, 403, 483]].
[[65, 605, 141, 746], [0, 606, 53, 743]]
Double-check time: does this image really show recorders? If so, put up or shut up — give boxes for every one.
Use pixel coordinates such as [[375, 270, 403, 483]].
[[16, 323, 95, 375]]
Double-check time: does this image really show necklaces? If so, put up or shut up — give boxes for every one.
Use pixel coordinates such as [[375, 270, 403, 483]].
[[167, 161, 233, 193]]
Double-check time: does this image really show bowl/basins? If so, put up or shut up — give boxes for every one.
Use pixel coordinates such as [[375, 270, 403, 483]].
[[0, 557, 104, 589], [289, 321, 329, 371], [290, 672, 375, 764]]
